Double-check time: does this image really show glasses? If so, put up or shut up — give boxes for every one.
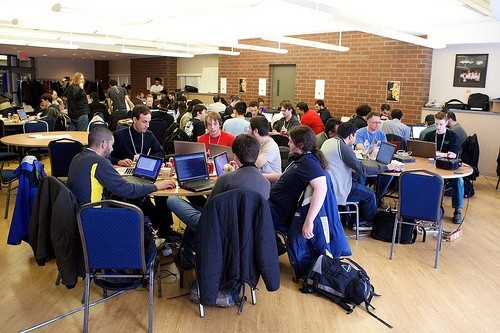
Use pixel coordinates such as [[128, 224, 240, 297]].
[[369, 121, 381, 124]]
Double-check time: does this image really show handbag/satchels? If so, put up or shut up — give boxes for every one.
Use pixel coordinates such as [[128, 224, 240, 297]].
[[369, 210, 426, 244], [299, 254, 382, 314], [444, 99, 469, 110], [188, 272, 247, 315], [468, 93, 490, 111], [443, 177, 475, 197]]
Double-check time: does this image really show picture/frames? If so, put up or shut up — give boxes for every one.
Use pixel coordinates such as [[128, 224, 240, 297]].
[[453, 54, 488, 88], [386, 81, 400, 102], [238, 78, 246, 94]]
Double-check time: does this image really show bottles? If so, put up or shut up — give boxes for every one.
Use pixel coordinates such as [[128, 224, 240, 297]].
[[223, 164, 231, 173], [363, 140, 370, 155], [7, 113, 11, 122]]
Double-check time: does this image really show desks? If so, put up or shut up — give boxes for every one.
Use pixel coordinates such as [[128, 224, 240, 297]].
[[420, 107, 500, 177], [0, 131, 90, 178], [357, 151, 473, 210], [0, 116, 42, 153], [112, 162, 218, 288]]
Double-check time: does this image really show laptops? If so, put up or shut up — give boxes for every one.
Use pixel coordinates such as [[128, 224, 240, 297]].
[[17, 109, 29, 121], [407, 125, 439, 158], [114, 141, 235, 192], [363, 141, 397, 167]]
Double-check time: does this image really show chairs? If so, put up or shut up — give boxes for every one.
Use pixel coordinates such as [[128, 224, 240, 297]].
[[0, 99, 478, 333]]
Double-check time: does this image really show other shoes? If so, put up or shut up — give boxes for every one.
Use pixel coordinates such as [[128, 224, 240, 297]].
[[453, 210, 462, 224], [352, 223, 373, 230], [377, 198, 384, 208], [156, 228, 183, 243]]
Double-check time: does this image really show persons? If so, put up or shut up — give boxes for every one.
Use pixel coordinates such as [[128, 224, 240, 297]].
[[419, 114, 437, 142], [315, 117, 343, 151], [185, 111, 236, 208], [260, 126, 328, 257], [0, 71, 332, 141], [247, 116, 282, 173], [66, 125, 177, 209], [378, 103, 391, 121], [354, 112, 395, 208], [348, 105, 372, 129], [424, 112, 465, 224], [446, 111, 469, 148], [107, 105, 183, 242], [319, 122, 403, 231], [381, 109, 411, 140], [166, 133, 272, 271]]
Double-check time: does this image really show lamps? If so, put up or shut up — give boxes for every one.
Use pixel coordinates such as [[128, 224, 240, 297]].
[[0, 0, 447, 58]]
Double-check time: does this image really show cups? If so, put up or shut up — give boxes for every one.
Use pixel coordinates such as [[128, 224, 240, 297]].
[[161, 168, 171, 180], [371, 144, 381, 160], [12, 114, 19, 122], [272, 129, 277, 135], [355, 145, 362, 156]]
